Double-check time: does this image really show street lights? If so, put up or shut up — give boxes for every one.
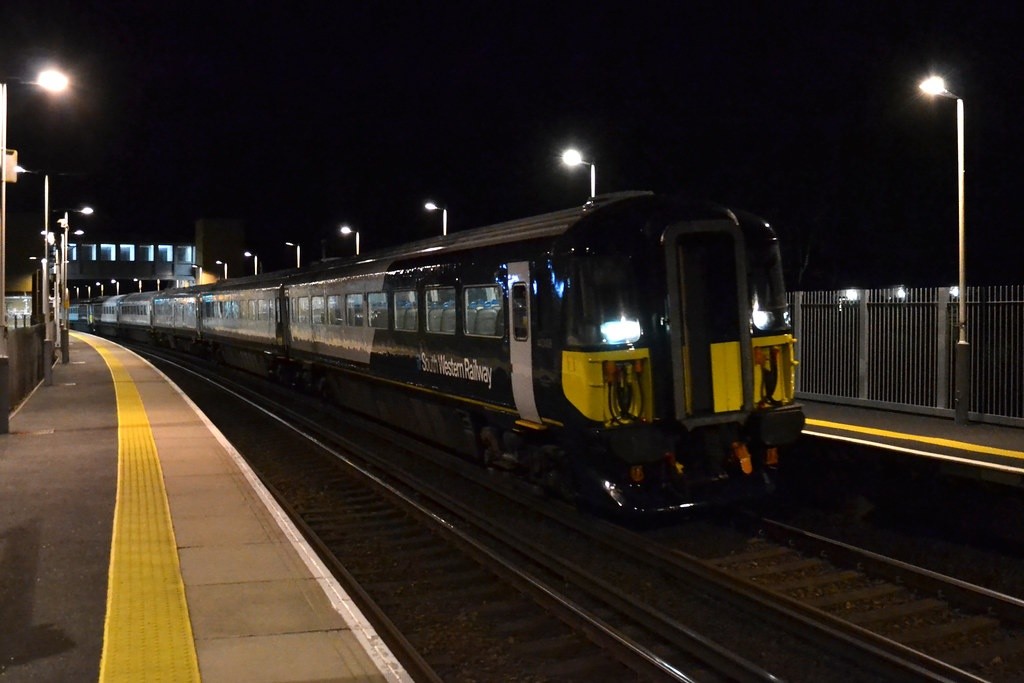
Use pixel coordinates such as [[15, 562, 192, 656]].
[[110, 280, 120, 295], [214, 260, 228, 281], [560, 148, 599, 197], [96, 282, 104, 296], [191, 264, 204, 285], [13, 165, 94, 345], [2, 71, 68, 347], [420, 200, 449, 238], [920, 76, 974, 424], [74, 286, 80, 299], [242, 252, 258, 275], [84, 285, 91, 298], [285, 241, 302, 268], [341, 225, 360, 254], [132, 277, 143, 293]]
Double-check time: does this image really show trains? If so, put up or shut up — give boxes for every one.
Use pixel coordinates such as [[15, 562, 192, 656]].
[[71, 189, 806, 524]]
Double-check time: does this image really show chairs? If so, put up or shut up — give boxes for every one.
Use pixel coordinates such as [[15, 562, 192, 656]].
[[299, 297, 505, 337]]
